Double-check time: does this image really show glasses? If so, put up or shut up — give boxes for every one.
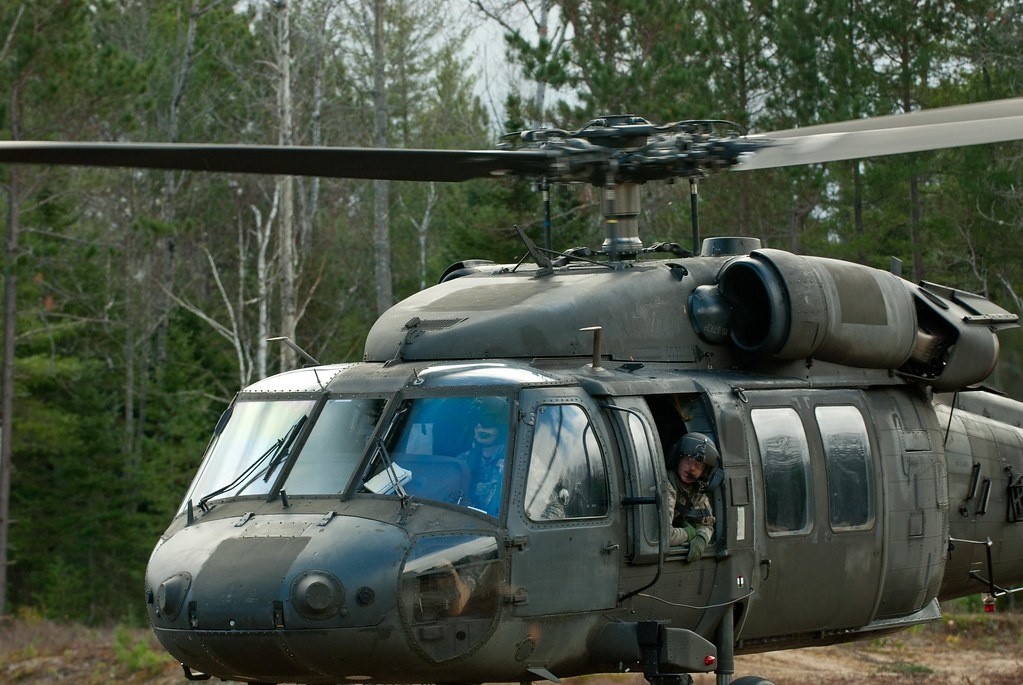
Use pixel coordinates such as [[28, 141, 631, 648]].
[[469, 414, 506, 429], [679, 454, 707, 470]]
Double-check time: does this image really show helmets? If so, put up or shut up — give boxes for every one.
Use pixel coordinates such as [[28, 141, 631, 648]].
[[468, 396, 510, 418], [676, 433, 721, 468]]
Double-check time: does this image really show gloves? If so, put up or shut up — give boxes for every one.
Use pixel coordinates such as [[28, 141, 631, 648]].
[[680, 518, 705, 567]]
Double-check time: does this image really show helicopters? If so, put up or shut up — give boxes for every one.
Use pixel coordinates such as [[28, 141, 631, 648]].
[[1, 110, 1023, 685]]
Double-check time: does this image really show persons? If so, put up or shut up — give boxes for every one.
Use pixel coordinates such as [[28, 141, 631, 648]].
[[455, 415, 572, 523], [662, 431, 724, 565]]
